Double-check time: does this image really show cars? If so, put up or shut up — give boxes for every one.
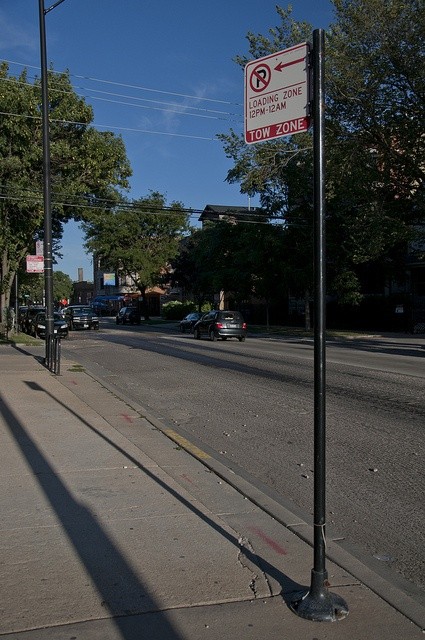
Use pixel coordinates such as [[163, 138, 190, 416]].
[[178, 313, 201, 333], [31, 312, 68, 340]]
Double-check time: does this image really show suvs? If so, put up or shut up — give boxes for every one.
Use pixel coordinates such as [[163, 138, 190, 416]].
[[65, 306, 99, 330], [193, 310, 247, 343], [115, 307, 141, 325], [19, 307, 28, 333], [25, 308, 45, 335]]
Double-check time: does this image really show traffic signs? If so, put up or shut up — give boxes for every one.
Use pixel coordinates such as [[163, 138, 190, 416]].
[[243, 41, 307, 145]]
